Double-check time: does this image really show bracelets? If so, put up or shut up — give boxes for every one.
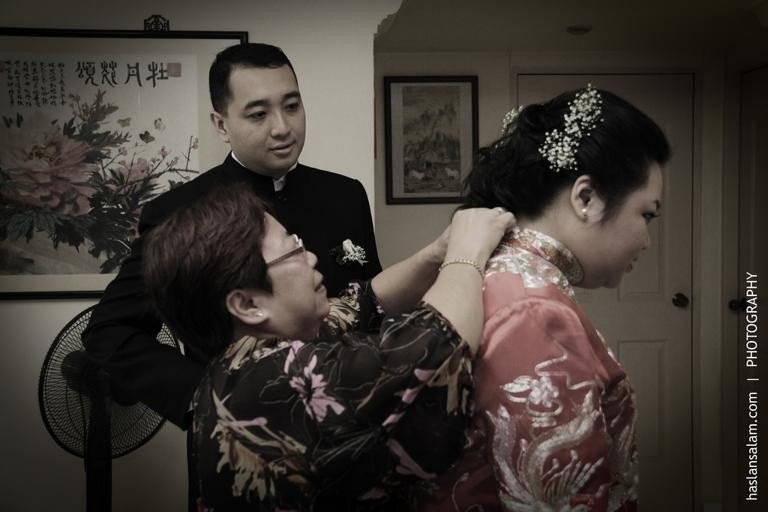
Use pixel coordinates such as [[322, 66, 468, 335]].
[[437, 258, 487, 291]]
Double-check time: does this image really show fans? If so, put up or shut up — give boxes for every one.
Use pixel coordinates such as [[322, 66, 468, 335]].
[[38, 302, 182, 512]]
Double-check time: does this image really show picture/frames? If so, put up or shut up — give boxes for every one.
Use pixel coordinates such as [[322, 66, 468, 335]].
[[0, 14, 249, 301], [383, 75, 479, 205]]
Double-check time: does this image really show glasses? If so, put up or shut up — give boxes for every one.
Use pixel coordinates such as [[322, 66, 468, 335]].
[[266, 234, 306, 267]]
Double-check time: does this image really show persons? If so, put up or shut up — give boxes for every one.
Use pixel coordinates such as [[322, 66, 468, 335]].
[[446, 80, 680, 511], [77, 43, 386, 510], [138, 180, 521, 510]]
